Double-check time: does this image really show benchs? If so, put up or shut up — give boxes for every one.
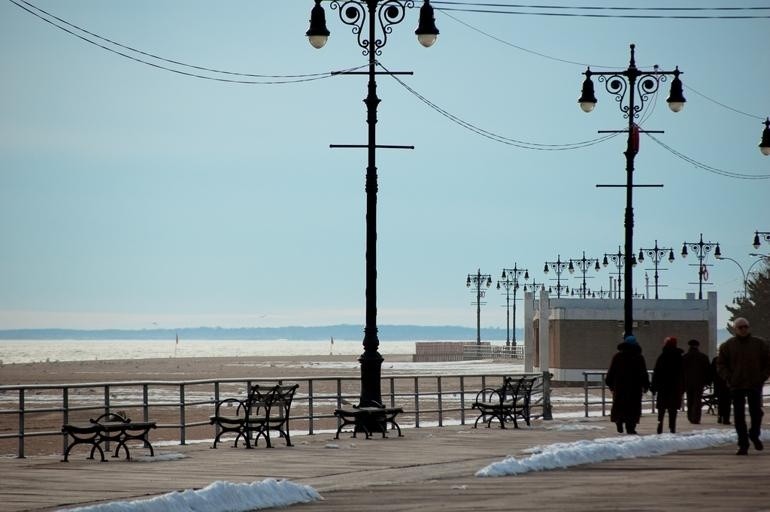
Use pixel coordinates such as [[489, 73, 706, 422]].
[[58, 412, 157, 462], [471, 375, 537, 430], [208, 382, 299, 449], [333, 398, 405, 441]]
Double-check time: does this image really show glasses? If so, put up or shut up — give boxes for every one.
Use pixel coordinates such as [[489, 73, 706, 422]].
[[738, 325, 748, 329]]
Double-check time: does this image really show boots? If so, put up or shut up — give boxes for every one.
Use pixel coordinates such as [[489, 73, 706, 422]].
[[657, 410, 665, 433], [667, 411, 677, 433]]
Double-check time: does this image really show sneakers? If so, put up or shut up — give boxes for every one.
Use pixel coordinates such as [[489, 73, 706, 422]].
[[750, 435, 763, 450], [718, 419, 722, 423], [617, 426, 623, 433], [625, 429, 637, 434], [724, 421, 730, 424], [735, 448, 748, 455]]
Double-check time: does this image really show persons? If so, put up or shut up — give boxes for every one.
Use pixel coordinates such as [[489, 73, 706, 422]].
[[651, 335, 686, 433], [718, 316, 770, 455], [710, 343, 732, 425], [683, 339, 710, 424], [605, 335, 649, 435]]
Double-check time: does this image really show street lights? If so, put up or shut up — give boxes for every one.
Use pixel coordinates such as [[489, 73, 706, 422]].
[[578, 43, 686, 344], [306, 0, 438, 434]]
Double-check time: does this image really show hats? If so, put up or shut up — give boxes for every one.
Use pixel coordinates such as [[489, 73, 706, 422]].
[[688, 340, 699, 345], [664, 336, 677, 347]]
